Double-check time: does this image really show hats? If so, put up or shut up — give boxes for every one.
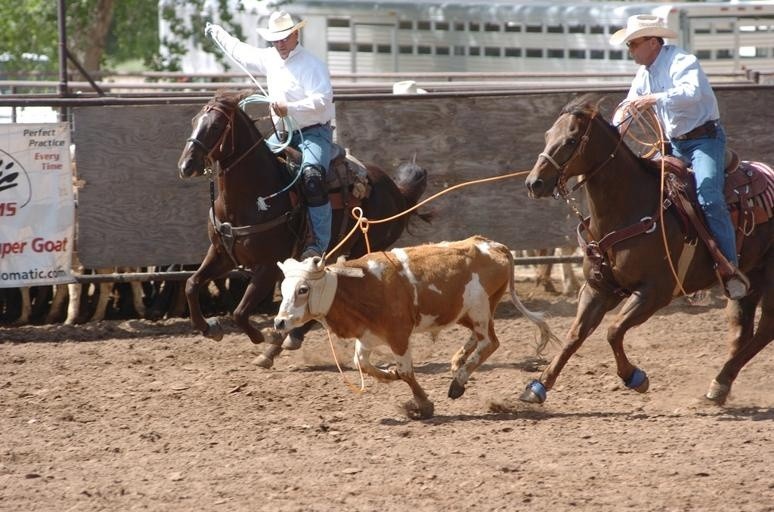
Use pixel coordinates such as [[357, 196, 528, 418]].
[[607, 13, 678, 49], [253, 12, 310, 44]]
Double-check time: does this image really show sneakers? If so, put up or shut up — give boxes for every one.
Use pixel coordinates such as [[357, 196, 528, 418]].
[[725, 275, 752, 303]]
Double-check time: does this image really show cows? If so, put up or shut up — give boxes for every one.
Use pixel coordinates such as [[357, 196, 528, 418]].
[[273, 234, 566, 420]]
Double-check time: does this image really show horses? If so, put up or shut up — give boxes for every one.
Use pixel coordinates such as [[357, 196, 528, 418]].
[[177, 85, 434, 369], [517, 92, 774, 407]]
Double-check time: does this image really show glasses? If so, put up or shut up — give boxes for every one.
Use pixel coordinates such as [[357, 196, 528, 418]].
[[273, 32, 296, 44], [625, 37, 649, 48]]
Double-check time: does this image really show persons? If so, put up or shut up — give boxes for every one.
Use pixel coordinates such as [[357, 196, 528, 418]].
[[202, 8, 336, 267], [607, 11, 749, 302]]
